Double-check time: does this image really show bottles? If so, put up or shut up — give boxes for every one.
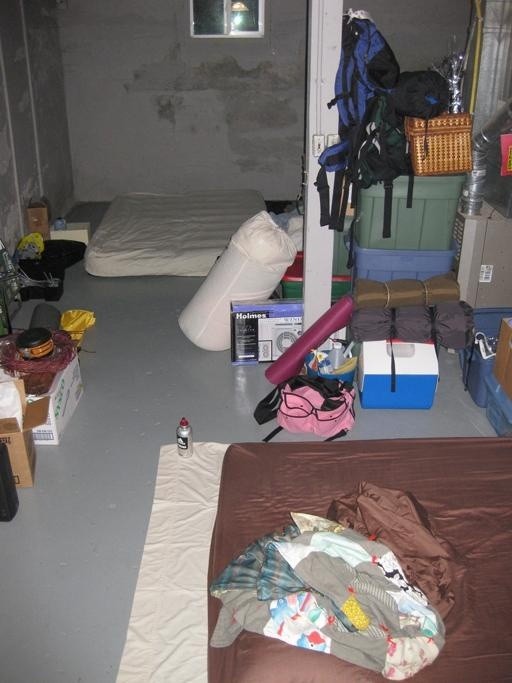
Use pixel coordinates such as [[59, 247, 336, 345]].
[[174, 414, 196, 458]]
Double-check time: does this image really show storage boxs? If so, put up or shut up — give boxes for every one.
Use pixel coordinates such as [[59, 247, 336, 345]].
[[0, 347, 84, 488]]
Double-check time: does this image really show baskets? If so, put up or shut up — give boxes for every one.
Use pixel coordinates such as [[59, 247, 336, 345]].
[[403, 112, 474, 177]]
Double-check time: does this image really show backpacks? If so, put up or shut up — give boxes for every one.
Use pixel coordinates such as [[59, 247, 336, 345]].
[[317, 4, 452, 190]]
